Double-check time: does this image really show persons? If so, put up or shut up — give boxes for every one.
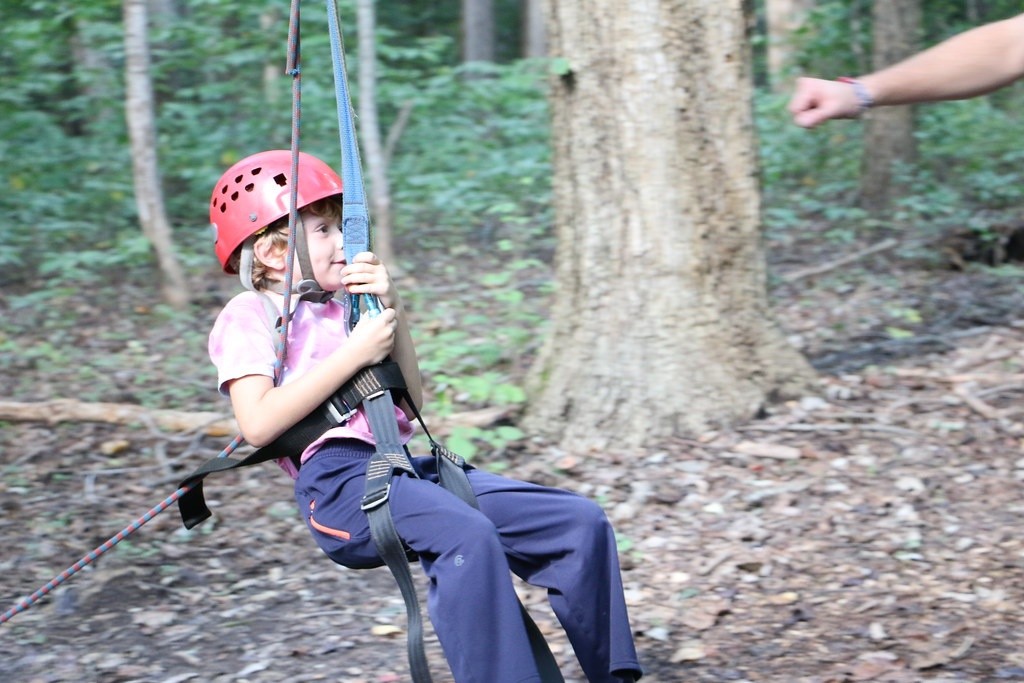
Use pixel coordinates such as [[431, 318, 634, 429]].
[[791, 14, 1024, 128], [207, 150, 642, 683]]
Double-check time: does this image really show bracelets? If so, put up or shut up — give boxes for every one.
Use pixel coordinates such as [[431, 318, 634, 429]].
[[838, 76, 872, 109]]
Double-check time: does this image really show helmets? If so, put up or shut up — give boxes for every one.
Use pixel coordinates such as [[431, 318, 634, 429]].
[[209, 150, 343, 274]]
